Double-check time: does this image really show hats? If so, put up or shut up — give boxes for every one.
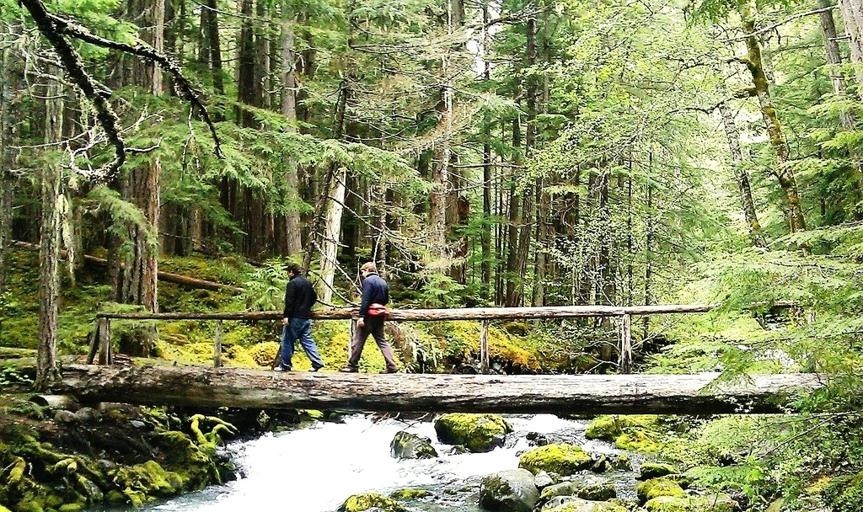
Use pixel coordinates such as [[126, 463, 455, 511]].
[[282, 263, 300, 271]]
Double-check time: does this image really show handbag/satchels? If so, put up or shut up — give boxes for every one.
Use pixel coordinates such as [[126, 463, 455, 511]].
[[368, 302, 387, 316]]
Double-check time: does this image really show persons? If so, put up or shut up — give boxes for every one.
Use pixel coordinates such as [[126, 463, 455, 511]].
[[339, 261, 399, 374], [273, 261, 326, 372]]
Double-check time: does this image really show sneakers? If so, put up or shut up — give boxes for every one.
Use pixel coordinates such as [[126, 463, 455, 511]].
[[339, 366, 358, 373], [274, 366, 291, 371], [379, 368, 398, 374], [308, 367, 316, 372]]
